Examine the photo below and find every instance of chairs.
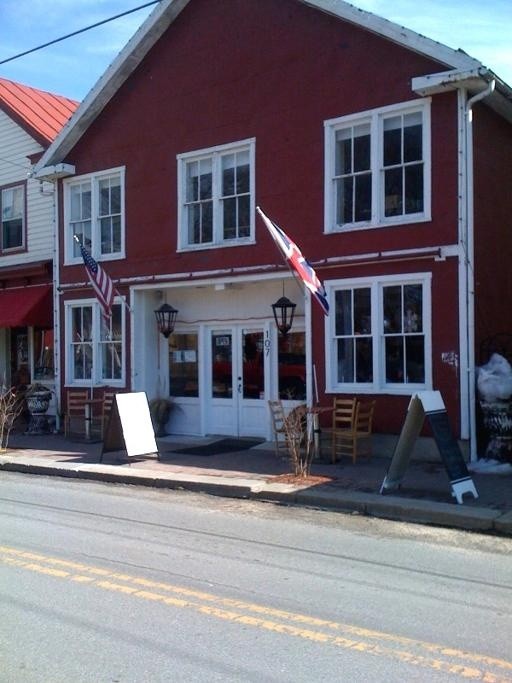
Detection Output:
[62,390,119,439]
[269,396,376,463]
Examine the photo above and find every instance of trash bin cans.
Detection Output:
[480,396,512,464]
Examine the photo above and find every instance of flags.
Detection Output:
[79,245,115,319]
[258,206,329,317]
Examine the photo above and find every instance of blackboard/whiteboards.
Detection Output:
[115,392,159,457]
[425,409,471,484]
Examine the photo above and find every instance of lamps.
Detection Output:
[272,293,295,332]
[155,302,178,337]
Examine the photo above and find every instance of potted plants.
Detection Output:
[150,399,176,438]
[24,382,52,434]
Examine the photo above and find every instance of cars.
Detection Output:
[213,345,306,399]
